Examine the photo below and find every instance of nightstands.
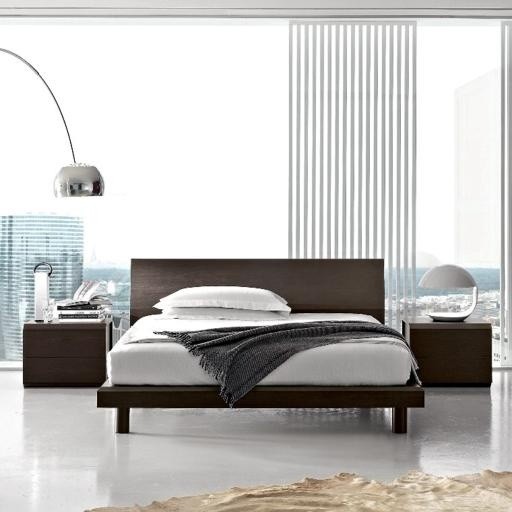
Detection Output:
[401,317,492,389]
[22,318,114,388]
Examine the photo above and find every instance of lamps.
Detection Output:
[0,48,104,199]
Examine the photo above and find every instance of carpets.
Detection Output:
[83,469,511,512]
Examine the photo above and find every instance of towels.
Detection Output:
[33,272,50,321]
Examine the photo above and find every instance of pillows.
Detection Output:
[151,285,293,322]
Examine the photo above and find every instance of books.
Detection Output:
[56,281,113,324]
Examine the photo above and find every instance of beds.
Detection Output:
[96,257,425,435]
